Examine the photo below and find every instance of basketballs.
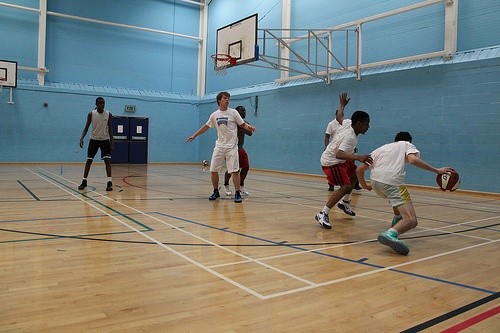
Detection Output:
[202,160,208,166]
[436,169,461,192]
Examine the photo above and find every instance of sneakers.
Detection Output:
[329,184,334,191]
[338,199,355,216]
[240,183,249,195]
[390,216,402,227]
[209,187,221,200]
[78,179,87,190]
[235,190,242,202]
[353,182,362,190]
[315,211,331,229]
[223,184,232,196]
[106,181,113,191]
[378,231,410,255]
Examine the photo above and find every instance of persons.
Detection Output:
[186,91,256,203]
[315,92,374,229]
[325,110,363,191]
[356,131,456,255]
[78,97,113,191]
[224,106,252,195]
